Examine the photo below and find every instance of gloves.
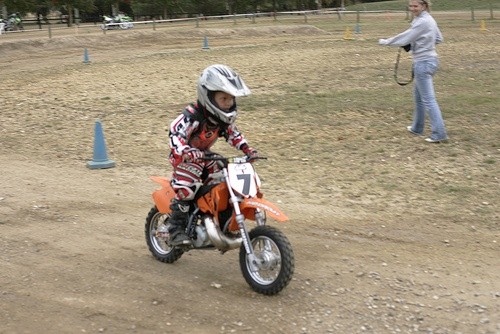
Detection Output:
[182,147,204,162]
[240,143,259,163]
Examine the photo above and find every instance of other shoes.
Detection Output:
[406,125,419,135]
[425,137,443,143]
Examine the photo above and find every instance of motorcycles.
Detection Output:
[145,152,294,296]
[2,17,24,32]
[101,12,133,30]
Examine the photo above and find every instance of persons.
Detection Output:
[169,64,259,246]
[378,0,450,142]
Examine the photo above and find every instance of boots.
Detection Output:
[167,199,192,246]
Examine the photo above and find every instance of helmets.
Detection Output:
[197,64,251,125]
